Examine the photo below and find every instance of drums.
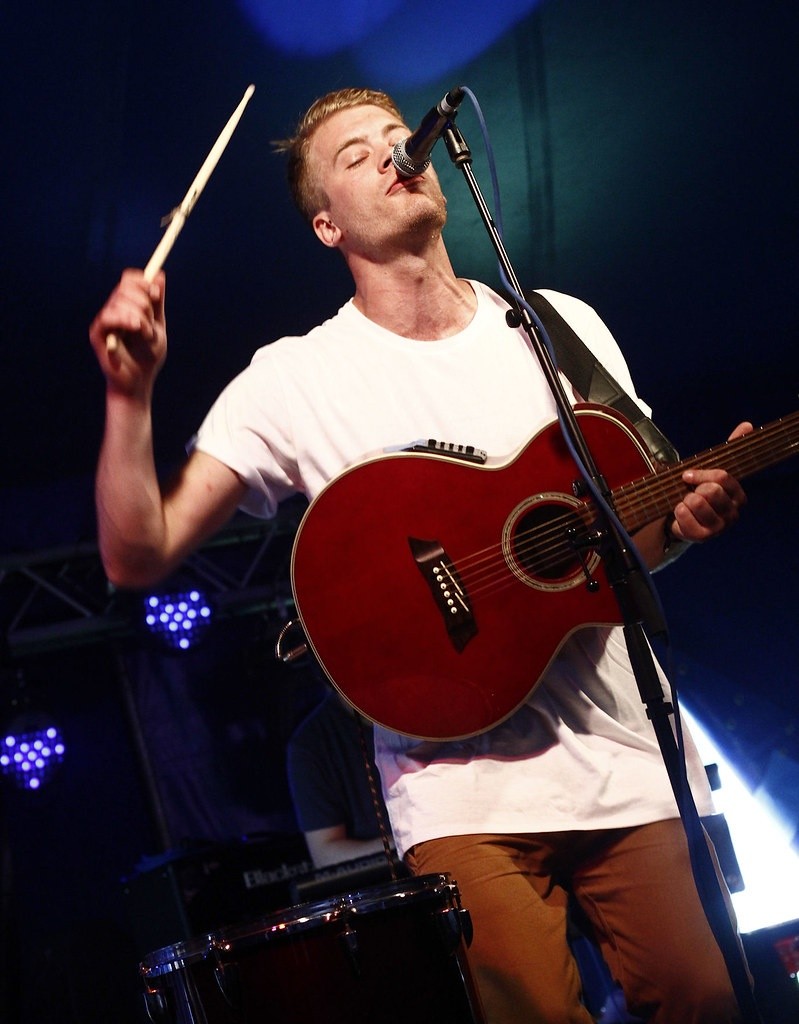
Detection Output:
[137,868,478,1023]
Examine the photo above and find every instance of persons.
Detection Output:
[87,88,754,1024]
[286,658,398,870]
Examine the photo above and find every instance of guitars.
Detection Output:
[288,398,799,743]
